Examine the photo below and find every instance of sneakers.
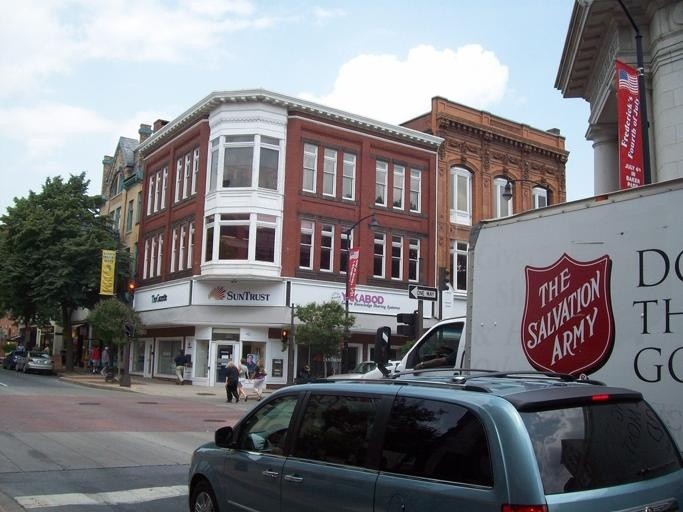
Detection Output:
[225,393,261,403]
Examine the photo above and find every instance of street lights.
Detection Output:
[341,211,377,373]
[502,177,551,208]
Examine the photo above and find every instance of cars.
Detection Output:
[348,360,400,374]
[2,350,24,369]
[15,350,55,374]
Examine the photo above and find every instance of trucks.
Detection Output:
[361,178,683,458]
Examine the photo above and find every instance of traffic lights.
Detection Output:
[127,279,135,298]
[438,265,449,293]
[125,324,133,338]
[395,311,416,335]
[280,330,286,341]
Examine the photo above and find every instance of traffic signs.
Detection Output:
[406,283,438,301]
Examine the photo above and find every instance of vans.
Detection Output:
[185,374,681,511]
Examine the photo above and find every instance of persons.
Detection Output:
[89,345,100,374]
[250,358,267,401]
[172,349,187,385]
[237,358,249,402]
[246,354,256,374]
[43,342,50,352]
[297,365,310,383]
[15,342,25,352]
[222,360,238,403]
[100,346,111,375]
